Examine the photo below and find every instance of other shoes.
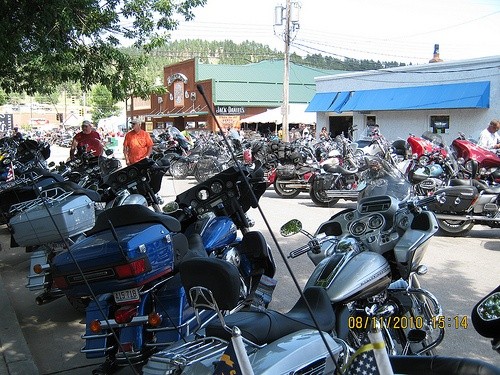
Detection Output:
[157,196,164,205]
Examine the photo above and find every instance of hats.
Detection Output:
[81,120,90,127]
[129,119,142,125]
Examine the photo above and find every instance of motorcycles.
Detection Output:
[0,127,500,375]
[51,160,278,375]
[143,155,446,375]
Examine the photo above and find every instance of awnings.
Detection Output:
[304,80,490,113]
[240,102,317,125]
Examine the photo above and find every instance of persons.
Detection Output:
[5,129,41,139]
[476,119,500,154]
[278,123,327,141]
[70,120,103,160]
[123,118,153,165]
